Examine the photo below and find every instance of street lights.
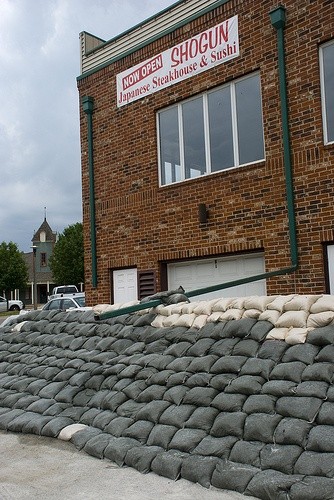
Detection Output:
[30,245,43,309]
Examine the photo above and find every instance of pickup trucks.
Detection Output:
[47,285,84,302]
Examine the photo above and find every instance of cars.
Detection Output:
[42,295,85,310]
[0,295,23,311]
